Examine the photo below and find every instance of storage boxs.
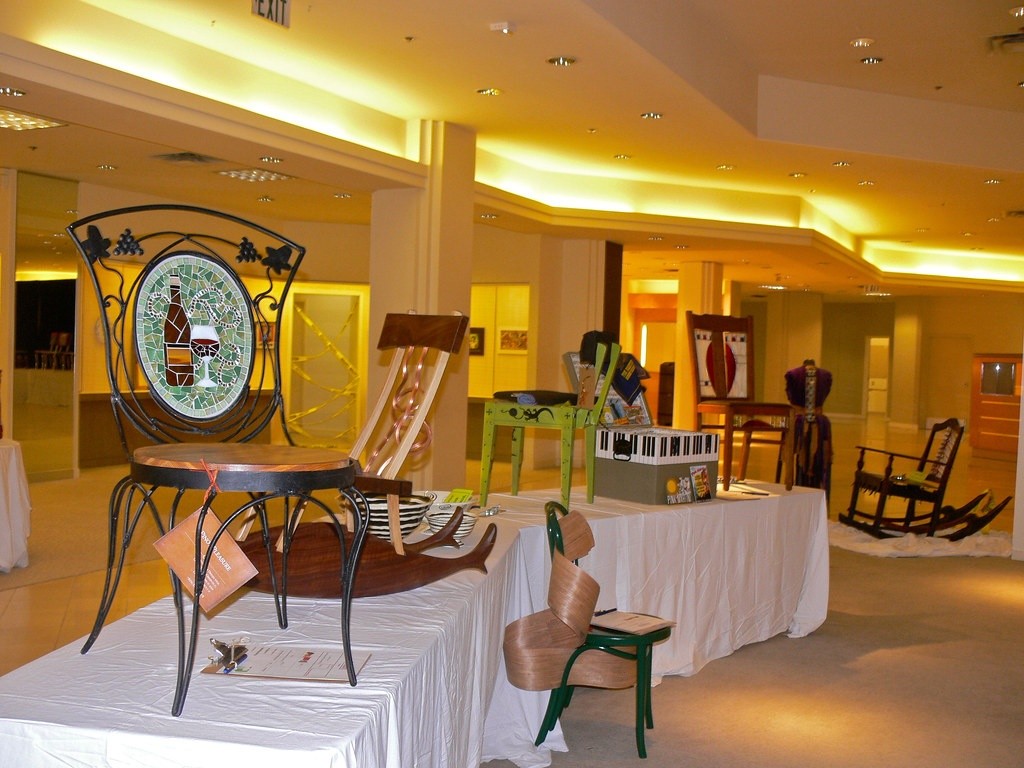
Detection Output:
[563,351,718,504]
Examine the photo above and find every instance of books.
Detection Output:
[690,465,712,504]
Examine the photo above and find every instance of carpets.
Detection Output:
[828,519,1014,557]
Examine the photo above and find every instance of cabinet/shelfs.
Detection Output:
[968,353,1023,463]
[658,362,673,425]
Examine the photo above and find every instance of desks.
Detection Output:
[13,368,73,409]
[0,436,831,768]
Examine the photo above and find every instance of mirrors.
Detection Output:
[981,362,1016,395]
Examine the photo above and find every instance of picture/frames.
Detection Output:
[470,327,485,355]
[254,321,275,349]
[497,327,528,355]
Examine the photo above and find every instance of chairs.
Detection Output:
[534,499,670,759]
[479,342,621,507]
[841,420,1013,540]
[66,204,371,722]
[227,308,498,601]
[34,331,73,369]
[685,309,798,490]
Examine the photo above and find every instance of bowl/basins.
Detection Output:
[425,511,478,548]
[346,493,436,542]
[412,491,479,517]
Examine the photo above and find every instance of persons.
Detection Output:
[784,359,832,487]
